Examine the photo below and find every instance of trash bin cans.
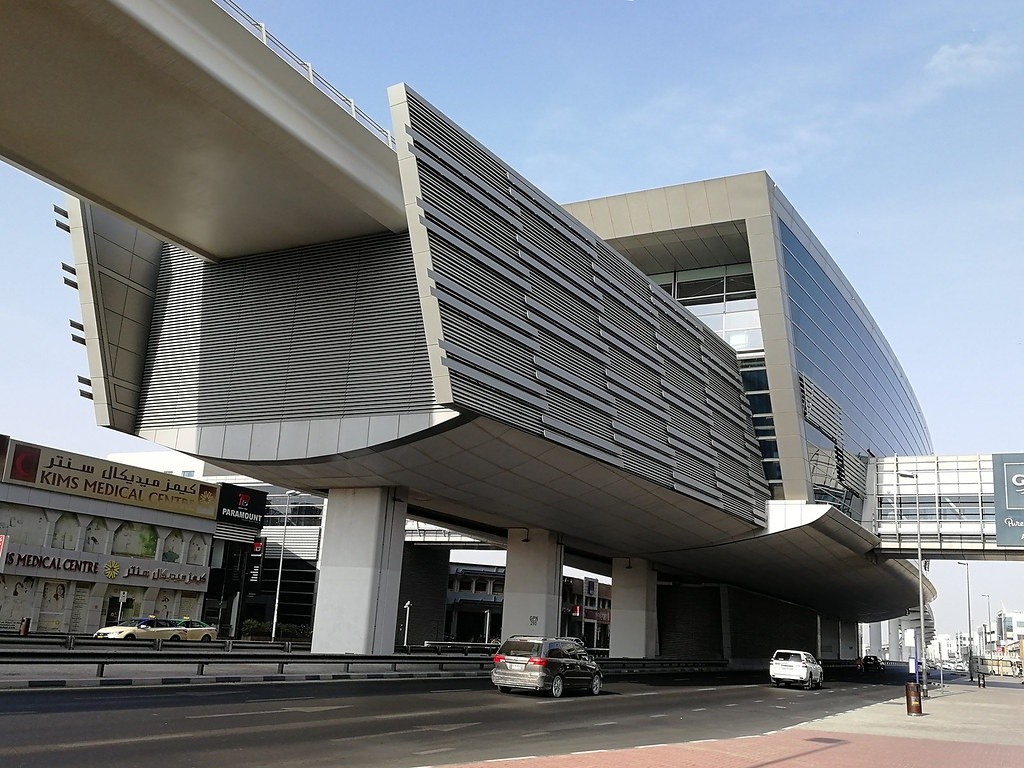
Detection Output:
[978,674,985,688]
[906,683,922,716]
[19,617,31,635]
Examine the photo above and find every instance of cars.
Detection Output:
[862,655,886,673]
[918,658,969,676]
[92,614,189,642]
[171,616,218,643]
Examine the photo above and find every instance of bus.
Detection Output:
[969,653,1023,678]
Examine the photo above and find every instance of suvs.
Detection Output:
[489,634,604,698]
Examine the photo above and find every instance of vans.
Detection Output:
[768,649,824,690]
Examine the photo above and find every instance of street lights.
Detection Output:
[269,489,301,641]
[894,470,929,700]
[957,561,973,682]
[982,594,994,676]
